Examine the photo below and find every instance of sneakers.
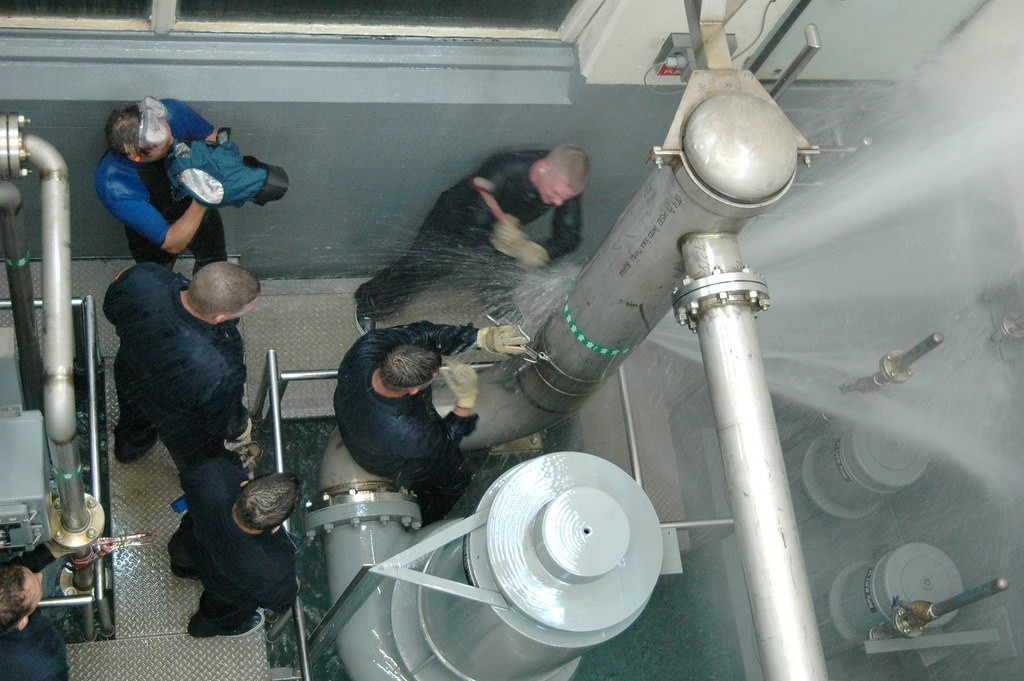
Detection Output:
[232,441,262,473]
[355,301,377,335]
[214,610,265,637]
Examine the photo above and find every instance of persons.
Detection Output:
[334,320,527,527]
[95,98,228,276]
[0,540,74,681]
[355,144,590,335]
[103,262,261,468]
[169,454,301,638]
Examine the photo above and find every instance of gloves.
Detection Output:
[477,325,526,356]
[442,359,479,408]
[44,536,75,558]
[491,212,549,271]
[224,417,252,452]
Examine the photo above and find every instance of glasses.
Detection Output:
[137,95,169,154]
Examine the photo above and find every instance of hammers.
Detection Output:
[471,175,508,223]
